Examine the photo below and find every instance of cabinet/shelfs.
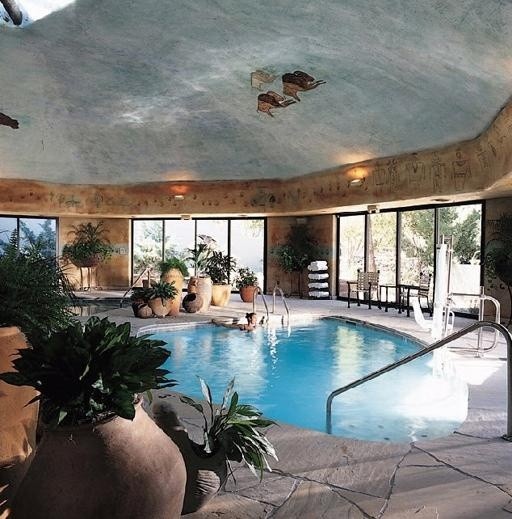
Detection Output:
[307,267,329,300]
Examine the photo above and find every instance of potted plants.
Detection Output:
[203,250,236,307]
[234,267,259,302]
[0,315,187,519]
[142,372,280,515]
[129,287,150,318]
[0,228,98,465]
[157,256,189,317]
[182,233,218,313]
[139,279,178,317]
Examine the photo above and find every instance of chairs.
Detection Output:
[347,269,382,309]
[400,272,432,317]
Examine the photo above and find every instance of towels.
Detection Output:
[307,260,330,299]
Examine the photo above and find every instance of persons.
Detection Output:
[211,311,260,333]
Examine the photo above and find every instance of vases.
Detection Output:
[183,292,203,312]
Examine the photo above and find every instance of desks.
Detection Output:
[379,285,418,314]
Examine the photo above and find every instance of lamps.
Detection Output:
[169,185,187,202]
[345,166,368,189]
